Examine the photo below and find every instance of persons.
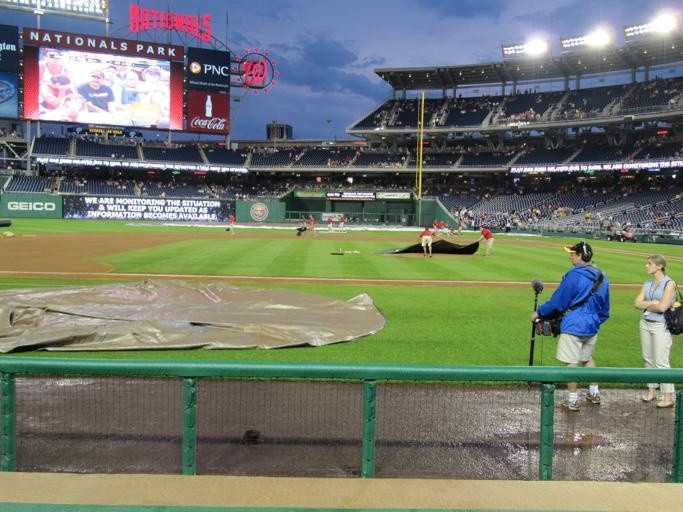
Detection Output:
[36,48,169,126]
[373,74,683,126]
[477,225,494,255]
[634,255,677,407]
[531,241,610,411]
[419,226,434,257]
[0,127,683,242]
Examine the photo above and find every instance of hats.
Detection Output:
[90,70,104,78]
[564,242,590,254]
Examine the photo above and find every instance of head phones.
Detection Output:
[581,242,591,262]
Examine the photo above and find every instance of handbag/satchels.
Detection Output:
[665,299,683,335]
[550,310,563,334]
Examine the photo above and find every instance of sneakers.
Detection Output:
[562,393,601,410]
[643,391,675,407]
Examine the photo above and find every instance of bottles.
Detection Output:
[205,95,212,117]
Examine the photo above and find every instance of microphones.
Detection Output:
[531,279,544,294]
